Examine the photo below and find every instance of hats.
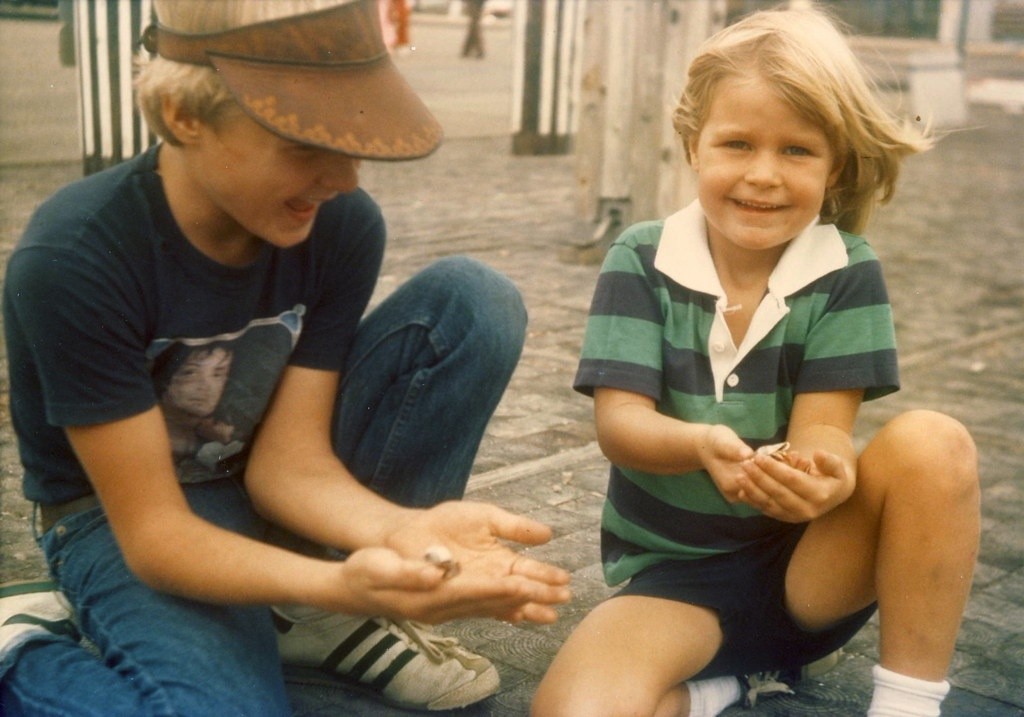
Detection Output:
[142,0,443,160]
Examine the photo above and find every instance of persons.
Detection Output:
[383,0,412,49]
[528,0,982,717]
[2,0,571,717]
[458,0,487,59]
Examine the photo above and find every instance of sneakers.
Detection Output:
[269,598,502,714]
[0,580,84,675]
[729,649,841,709]
